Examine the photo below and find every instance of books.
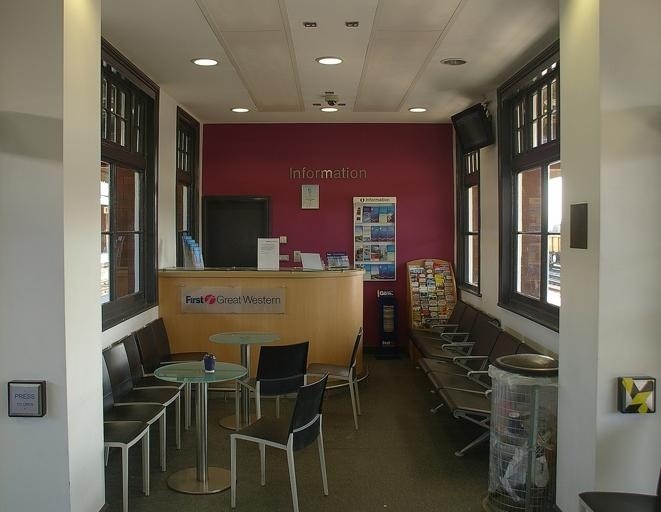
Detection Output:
[298,251,323,272]
[354,204,396,280]
[179,229,202,266]
[325,250,350,270]
[409,260,456,329]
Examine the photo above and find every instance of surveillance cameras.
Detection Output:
[326,96,342,106]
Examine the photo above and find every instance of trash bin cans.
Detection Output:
[482,354,559,512]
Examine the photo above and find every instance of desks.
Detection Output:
[207,331,279,430]
[151,361,248,495]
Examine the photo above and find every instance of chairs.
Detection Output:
[103,319,208,511]
[577,458,661,512]
[241,338,308,418]
[228,371,332,510]
[304,329,367,429]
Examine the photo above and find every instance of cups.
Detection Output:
[202,352,217,374]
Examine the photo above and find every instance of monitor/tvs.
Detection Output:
[450,102,495,153]
[202,194,271,266]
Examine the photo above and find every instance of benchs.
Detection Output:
[408,297,501,396]
[417,321,547,458]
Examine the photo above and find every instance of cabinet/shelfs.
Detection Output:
[407,257,458,330]
